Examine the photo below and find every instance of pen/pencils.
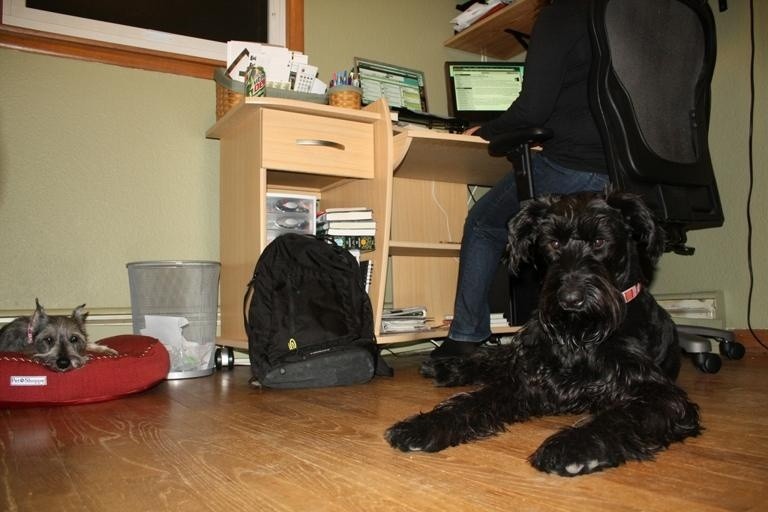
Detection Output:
[330,68,360,87]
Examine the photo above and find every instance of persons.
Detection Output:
[428,0,611,366]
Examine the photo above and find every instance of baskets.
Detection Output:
[328,85,361,110]
[213,66,327,121]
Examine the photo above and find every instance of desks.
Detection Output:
[377,120,543,347]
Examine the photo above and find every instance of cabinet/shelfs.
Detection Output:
[204,92,396,351]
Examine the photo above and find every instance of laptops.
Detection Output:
[354,56,461,123]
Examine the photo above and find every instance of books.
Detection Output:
[315,204,380,250]
[383,304,511,337]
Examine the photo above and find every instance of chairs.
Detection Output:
[489,1,746,375]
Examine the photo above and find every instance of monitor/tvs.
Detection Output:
[444,61,526,128]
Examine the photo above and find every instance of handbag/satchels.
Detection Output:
[243,232,393,389]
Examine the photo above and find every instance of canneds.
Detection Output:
[246,66,266,97]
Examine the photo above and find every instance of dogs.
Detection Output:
[384,182,706,477]
[0,299,120,373]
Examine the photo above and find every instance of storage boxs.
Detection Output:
[263,191,319,252]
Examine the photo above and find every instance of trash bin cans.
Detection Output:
[126,263,218,381]
